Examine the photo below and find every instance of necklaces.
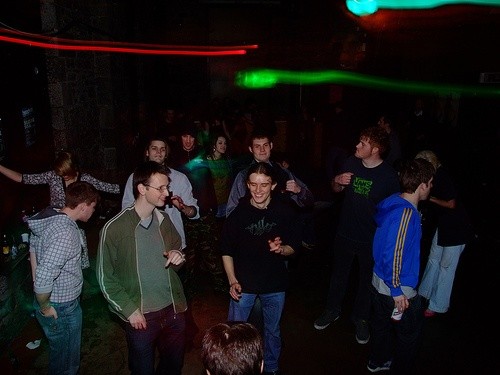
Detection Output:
[251,198,267,210]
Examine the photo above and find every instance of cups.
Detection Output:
[20,233,29,242]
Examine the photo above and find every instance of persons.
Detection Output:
[377,116,402,165]
[120,136,200,252]
[226,131,315,332]
[0,151,120,270]
[153,108,185,148]
[170,127,234,309]
[96,161,189,375]
[219,162,305,375]
[313,126,402,345]
[27,180,101,375]
[413,150,471,318]
[201,321,264,375]
[366,157,436,373]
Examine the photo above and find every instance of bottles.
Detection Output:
[11,233,17,260]
[391,307,402,321]
[2,234,10,257]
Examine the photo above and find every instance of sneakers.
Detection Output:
[355,319,371,344]
[366,357,394,372]
[313,310,341,330]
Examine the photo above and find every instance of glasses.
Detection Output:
[247,182,269,191]
[144,184,171,193]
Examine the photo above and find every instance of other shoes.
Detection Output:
[205,276,230,296]
[422,302,427,306]
[179,275,193,301]
[423,309,436,319]
